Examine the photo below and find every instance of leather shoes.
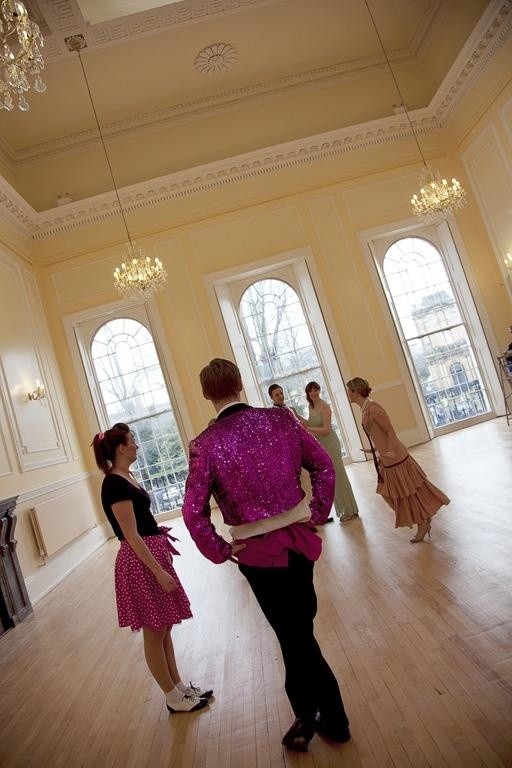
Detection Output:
[328,517,333,522]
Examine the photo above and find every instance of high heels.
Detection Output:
[409,524,431,543]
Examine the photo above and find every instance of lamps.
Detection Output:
[362,1,468,227]
[64,34,170,303]
[28,378,46,403]
[0,0,49,114]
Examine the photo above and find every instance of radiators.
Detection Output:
[29,485,97,557]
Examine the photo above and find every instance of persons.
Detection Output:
[182,359,351,751]
[268,384,333,523]
[290,382,358,522]
[346,377,450,543]
[93,423,213,712]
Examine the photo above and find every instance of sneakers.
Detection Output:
[282,718,316,751]
[315,713,350,744]
[166,695,208,712]
[185,687,213,699]
